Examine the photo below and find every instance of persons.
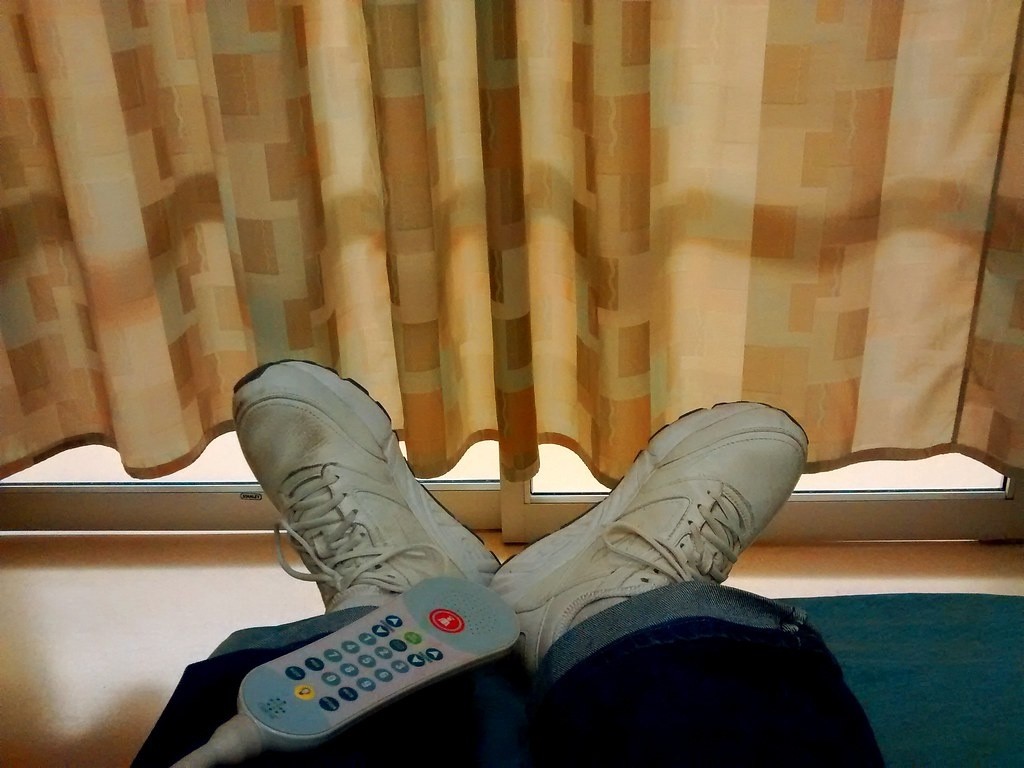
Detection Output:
[130,359,885,768]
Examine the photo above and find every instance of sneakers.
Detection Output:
[490,397,812,683]
[231,357,500,602]
